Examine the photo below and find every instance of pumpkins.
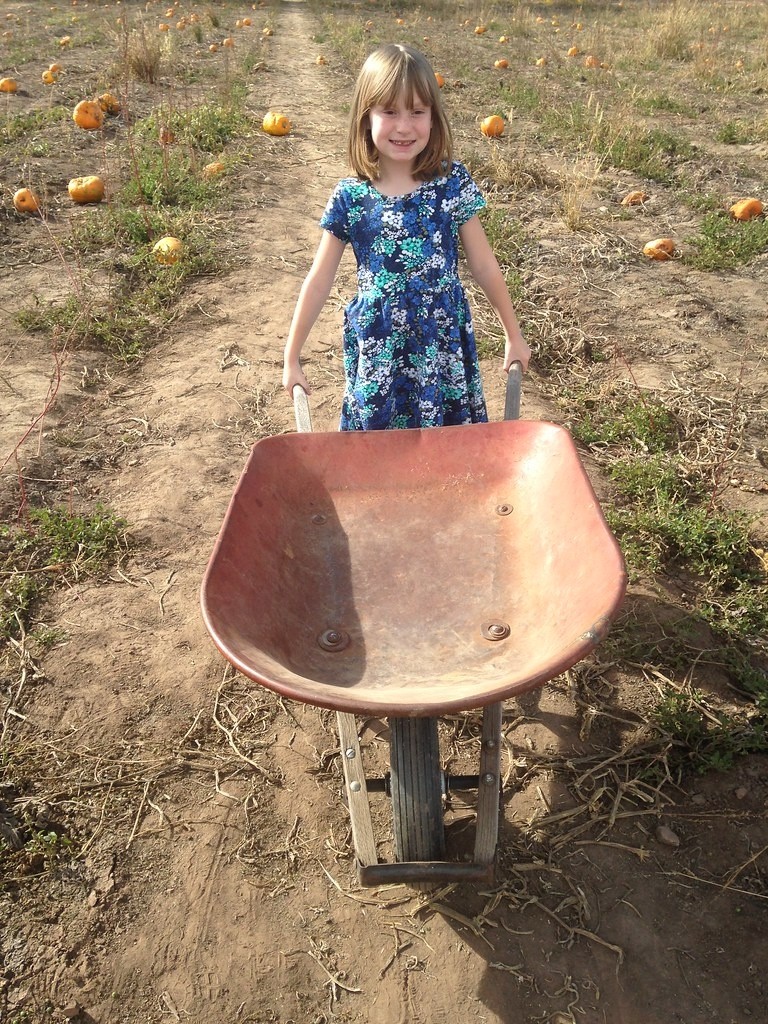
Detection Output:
[0,0,763,264]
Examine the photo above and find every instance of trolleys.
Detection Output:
[196,353,636,891]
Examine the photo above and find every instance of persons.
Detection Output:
[281,44,532,431]
